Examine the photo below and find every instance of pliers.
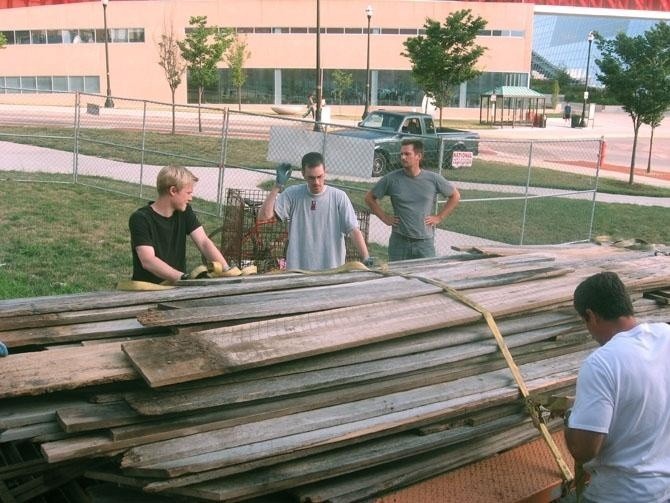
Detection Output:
[513,394,574,417]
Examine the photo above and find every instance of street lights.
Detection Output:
[362,4,374,119]
[580,29,595,127]
[100,0,114,109]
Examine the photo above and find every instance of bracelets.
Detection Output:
[563,406,574,423]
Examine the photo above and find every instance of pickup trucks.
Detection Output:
[327,109,481,178]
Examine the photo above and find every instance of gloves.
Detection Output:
[361,256,374,266]
[180,273,210,280]
[276,162,292,188]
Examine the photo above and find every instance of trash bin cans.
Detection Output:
[571,114,580,127]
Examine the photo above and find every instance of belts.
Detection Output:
[392,232,424,241]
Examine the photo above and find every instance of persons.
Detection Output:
[563,100,572,128]
[302,92,315,120]
[561,272,670,503]
[365,138,460,261]
[257,151,373,273]
[128,162,230,285]
